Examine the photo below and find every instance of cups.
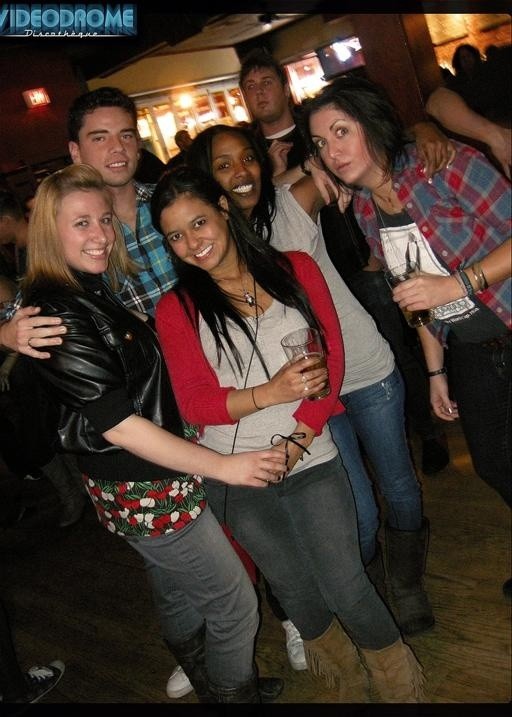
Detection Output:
[280,327,332,402]
[385,260,436,329]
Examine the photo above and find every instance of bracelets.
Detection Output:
[250,384,264,409]
[458,271,474,297]
[479,267,489,290]
[425,368,446,377]
[451,272,466,298]
[471,265,483,291]
[270,432,309,462]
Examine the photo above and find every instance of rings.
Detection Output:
[303,382,308,390]
[299,372,306,381]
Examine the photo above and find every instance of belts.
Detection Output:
[471,331,511,352]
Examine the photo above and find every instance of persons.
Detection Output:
[162,36,511,176]
[0,631,73,705]
[181,117,457,637]
[18,162,294,707]
[240,51,450,478]
[2,86,355,699]
[144,165,434,705]
[306,74,512,602]
[0,166,91,530]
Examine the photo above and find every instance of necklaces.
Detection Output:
[219,270,263,307]
[367,184,400,214]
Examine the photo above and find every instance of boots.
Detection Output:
[39,450,90,530]
[163,618,284,704]
[356,637,428,704]
[300,614,371,704]
[384,514,436,638]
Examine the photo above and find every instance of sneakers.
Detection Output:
[280,617,309,671]
[166,663,194,699]
[22,658,67,704]
[421,428,450,473]
